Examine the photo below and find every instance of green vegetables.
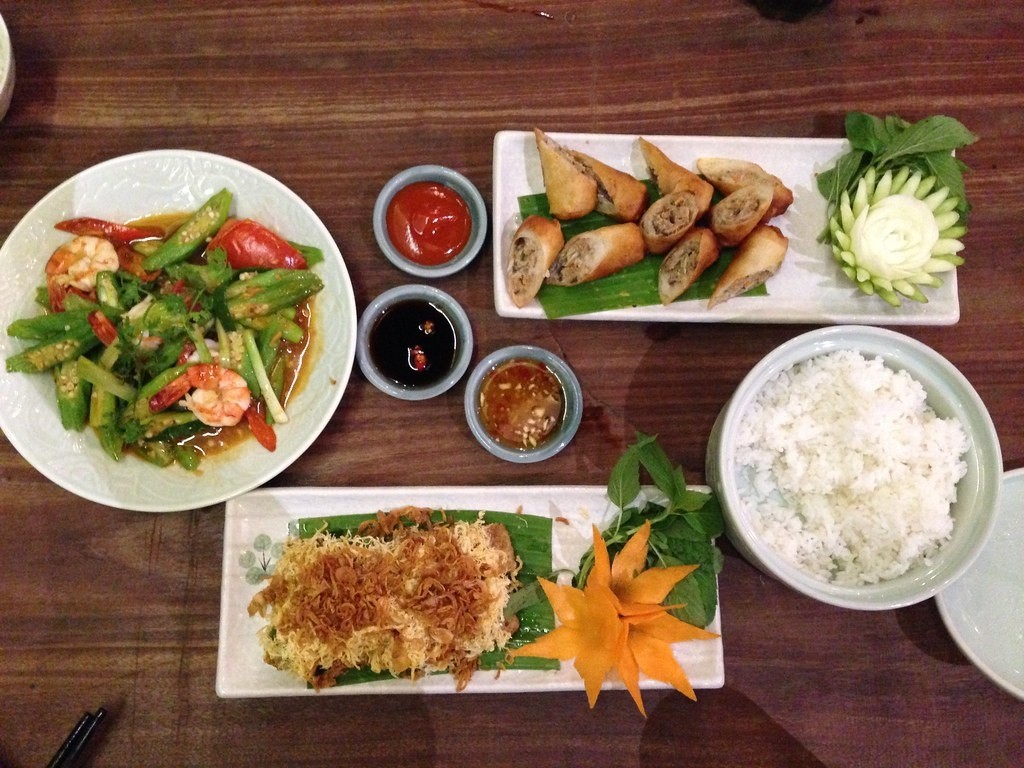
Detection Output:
[816,111,975,247]
[575,433,726,631]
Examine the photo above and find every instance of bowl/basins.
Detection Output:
[705,325,1003,609]
[357,285,473,399]
[374,165,487,276]
[463,346,584,463]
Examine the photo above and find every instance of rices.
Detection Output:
[730,348,971,587]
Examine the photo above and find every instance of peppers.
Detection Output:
[2,187,326,470]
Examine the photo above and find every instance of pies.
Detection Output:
[507,127,795,312]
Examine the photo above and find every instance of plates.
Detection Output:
[217,484,726,696]
[1,149,358,512]
[492,131,959,326]
[934,468,1023,700]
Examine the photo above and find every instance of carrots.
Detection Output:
[508,519,723,718]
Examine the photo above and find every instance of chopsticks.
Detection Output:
[46,707,105,768]
